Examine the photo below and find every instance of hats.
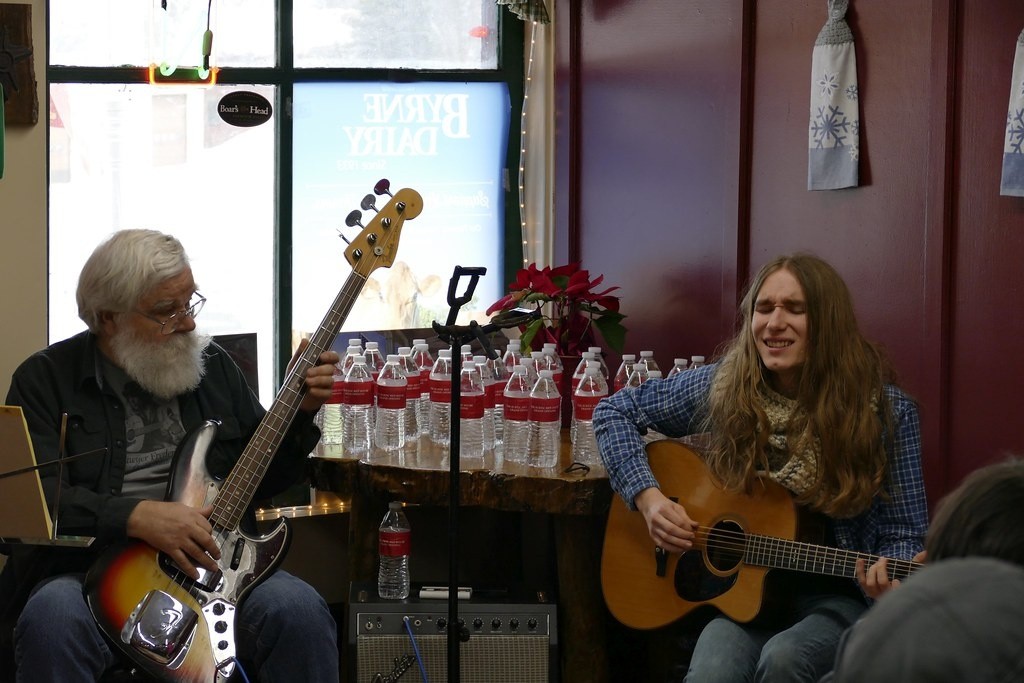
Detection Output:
[836,558,1024,683]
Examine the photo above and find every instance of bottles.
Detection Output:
[378,502,410,599]
[300,331,708,466]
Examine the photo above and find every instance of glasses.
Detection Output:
[135,292,207,335]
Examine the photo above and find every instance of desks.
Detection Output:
[304,426,613,683]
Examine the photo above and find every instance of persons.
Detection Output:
[592,252,928,683]
[5,229,340,683]
[830,458,1024,683]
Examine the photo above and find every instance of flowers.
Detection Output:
[487,263,630,355]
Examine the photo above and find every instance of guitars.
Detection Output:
[601,439,929,633]
[83,177,424,683]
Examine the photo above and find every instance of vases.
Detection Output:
[562,351,607,426]
